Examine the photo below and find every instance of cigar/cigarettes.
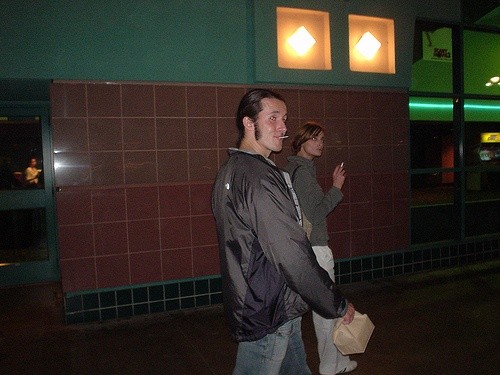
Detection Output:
[340,162,344,167]
[279,136,288,138]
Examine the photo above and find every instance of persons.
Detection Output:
[285,122,357,375]
[25,159,42,190]
[211,89,355,375]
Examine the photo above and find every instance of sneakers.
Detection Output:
[320,360,357,375]
